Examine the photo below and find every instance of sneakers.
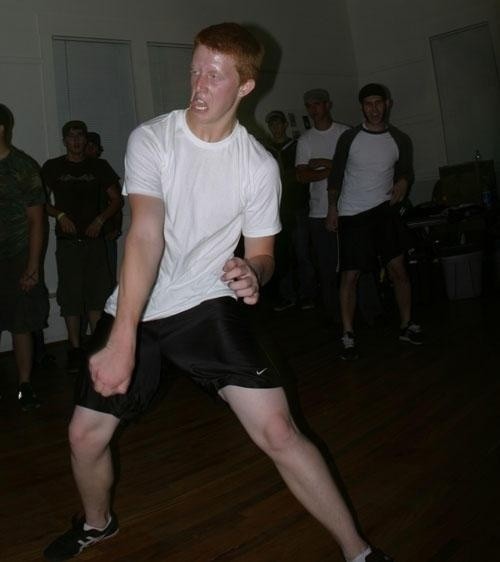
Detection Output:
[399,321,424,345]
[341,331,358,361]
[43,507,120,562]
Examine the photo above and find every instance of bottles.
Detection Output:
[479,175,492,209]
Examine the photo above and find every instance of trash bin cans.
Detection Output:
[438,245,485,304]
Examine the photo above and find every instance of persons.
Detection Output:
[39,121,122,369]
[83,130,125,286]
[327,84,433,365]
[43,23,402,561]
[1,101,49,403]
[291,85,397,338]
[264,104,316,312]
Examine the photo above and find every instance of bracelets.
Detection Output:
[97,216,102,225]
[56,211,64,219]
